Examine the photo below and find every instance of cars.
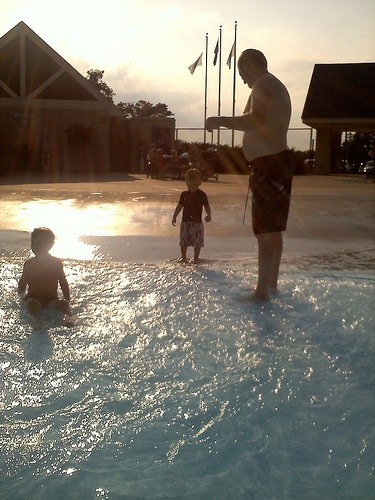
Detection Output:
[305,156,375,177]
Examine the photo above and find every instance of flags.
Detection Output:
[211,37,219,66]
[226,40,235,69]
[188,51,203,74]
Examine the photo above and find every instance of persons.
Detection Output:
[205,47,294,307]
[15,225,76,330]
[141,147,213,182]
[170,166,213,266]
